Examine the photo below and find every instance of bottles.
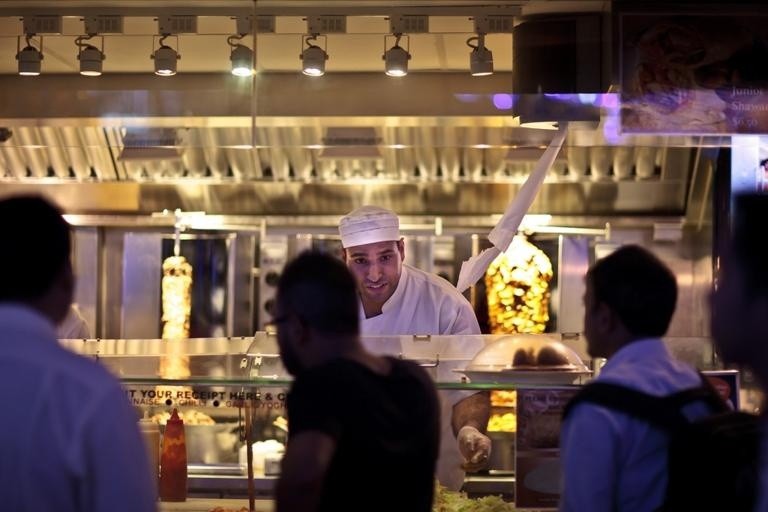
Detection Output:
[136,405,189,506]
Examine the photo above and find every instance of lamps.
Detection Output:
[505,127,570,163]
[317,126,383,160]
[114,127,184,162]
[13,26,494,79]
[152,225,202,406]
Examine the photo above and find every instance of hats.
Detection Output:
[339,206,401,248]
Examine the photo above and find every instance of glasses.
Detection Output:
[263,320,284,337]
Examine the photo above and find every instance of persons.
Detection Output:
[339,205,491,491]
[274,250,439,512]
[1,192,157,511]
[53,306,89,339]
[558,243,729,512]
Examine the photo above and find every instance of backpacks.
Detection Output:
[562,370,762,512]
